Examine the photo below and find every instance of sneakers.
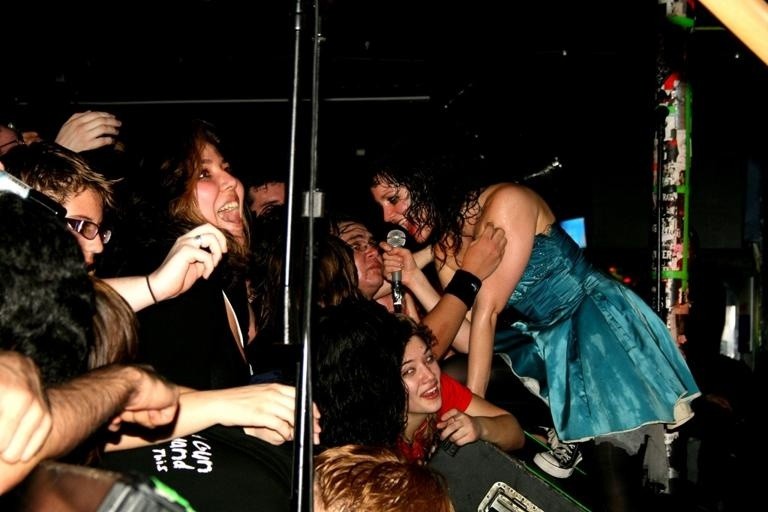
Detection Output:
[665,429,679,479]
[534,428,583,479]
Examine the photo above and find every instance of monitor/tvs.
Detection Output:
[559,217,587,249]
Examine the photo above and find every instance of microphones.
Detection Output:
[386,229,407,314]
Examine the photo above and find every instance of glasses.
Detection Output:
[0,138,24,147]
[66,217,112,245]
[351,240,379,254]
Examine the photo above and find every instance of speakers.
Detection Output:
[422,435,611,512]
